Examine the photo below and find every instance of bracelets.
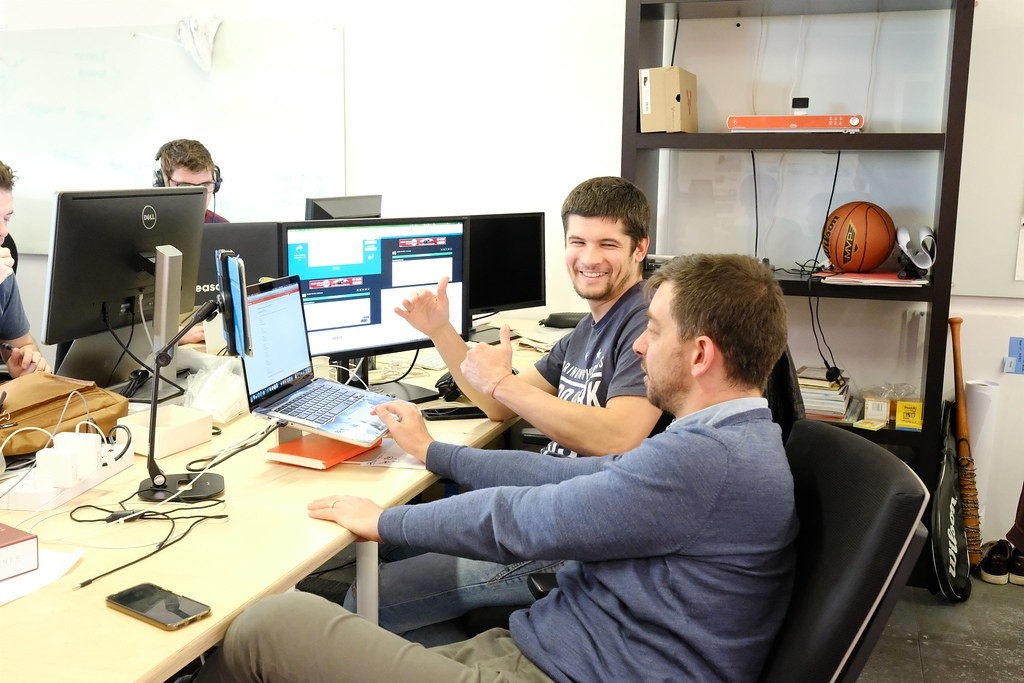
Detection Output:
[492,373,512,399]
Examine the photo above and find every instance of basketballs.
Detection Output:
[822,201,897,272]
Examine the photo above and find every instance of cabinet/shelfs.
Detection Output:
[619,0,977,584]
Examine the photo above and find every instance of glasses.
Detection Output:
[166,174,216,193]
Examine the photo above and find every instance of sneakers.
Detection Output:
[979,538,1024,586]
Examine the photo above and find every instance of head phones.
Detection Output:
[151,142,222,194]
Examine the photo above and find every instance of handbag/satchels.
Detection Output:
[0,369,129,456]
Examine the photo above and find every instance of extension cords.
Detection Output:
[0,444,135,511]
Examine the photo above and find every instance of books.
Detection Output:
[796,365,864,424]
[267,432,383,470]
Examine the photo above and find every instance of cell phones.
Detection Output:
[105,582,211,631]
[421,406,488,421]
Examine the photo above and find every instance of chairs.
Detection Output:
[756,420,932,683]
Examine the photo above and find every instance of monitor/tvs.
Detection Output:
[40,186,548,405]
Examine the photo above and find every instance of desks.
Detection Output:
[0,308,582,682]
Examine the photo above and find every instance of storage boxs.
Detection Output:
[639,68,697,132]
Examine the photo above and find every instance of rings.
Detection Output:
[330,500,341,508]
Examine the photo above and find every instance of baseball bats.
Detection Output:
[948,315,983,565]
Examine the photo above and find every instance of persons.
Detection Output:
[153,139,231,345]
[342,175,675,635]
[0,163,52,379]
[174,253,800,683]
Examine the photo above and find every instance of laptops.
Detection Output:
[56,319,154,388]
[243,274,409,447]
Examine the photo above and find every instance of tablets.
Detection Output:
[213,248,256,360]
